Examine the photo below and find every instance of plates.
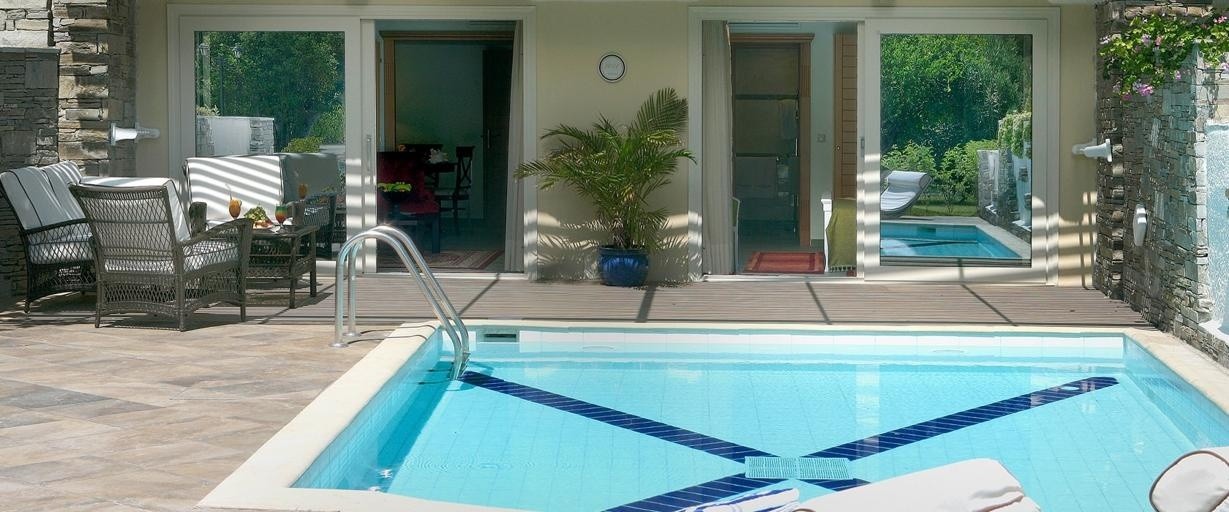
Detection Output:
[253,224,274,230]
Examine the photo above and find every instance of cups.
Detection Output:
[298,184,307,201]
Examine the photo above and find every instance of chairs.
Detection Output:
[879,170,933,219]
[70,155,286,331]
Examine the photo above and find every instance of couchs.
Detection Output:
[0,160,98,312]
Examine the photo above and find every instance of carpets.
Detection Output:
[742,251,825,274]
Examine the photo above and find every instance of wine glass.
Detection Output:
[229,199,241,220]
[275,204,288,232]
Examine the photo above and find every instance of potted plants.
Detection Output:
[513,88,699,286]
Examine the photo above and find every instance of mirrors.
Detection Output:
[878,34,1033,268]
